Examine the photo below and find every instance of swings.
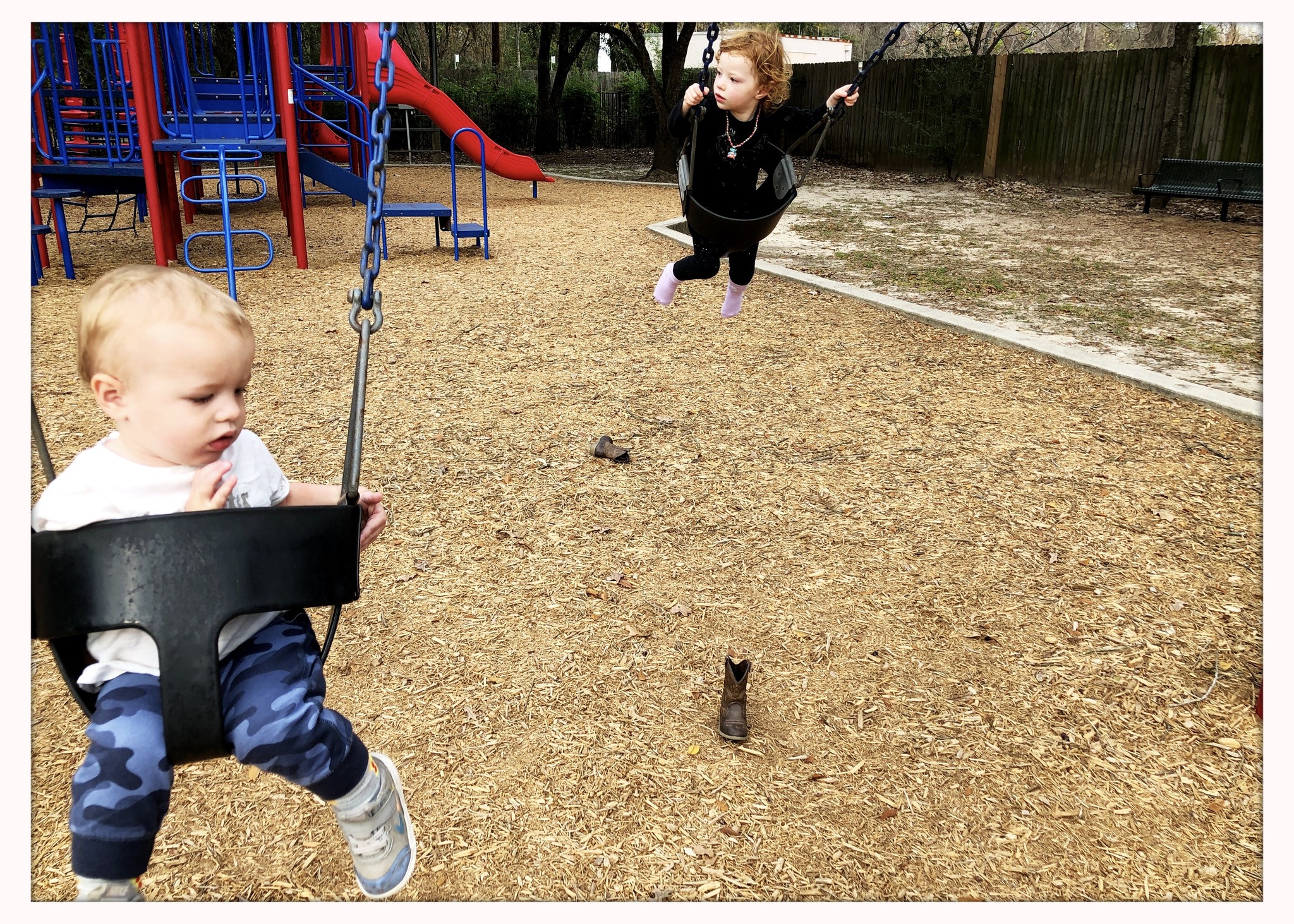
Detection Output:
[676,21,910,252]
[33,23,399,763]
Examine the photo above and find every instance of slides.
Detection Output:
[292,23,557,182]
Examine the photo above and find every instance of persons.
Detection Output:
[654,22,859,319]
[31,265,417,902]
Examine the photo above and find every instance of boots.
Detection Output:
[718,655,752,741]
[590,435,631,463]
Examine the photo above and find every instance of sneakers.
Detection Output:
[76,880,146,902]
[331,752,416,898]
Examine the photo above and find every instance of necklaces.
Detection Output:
[726,105,761,160]
